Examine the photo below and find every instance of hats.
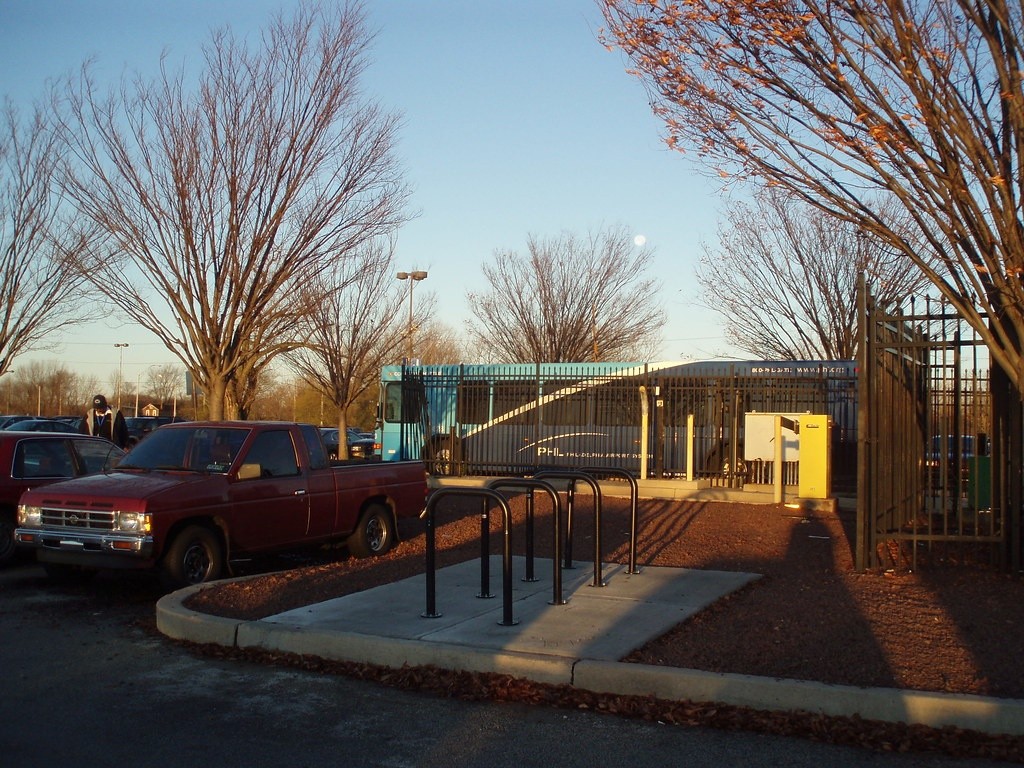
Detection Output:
[92,394,107,408]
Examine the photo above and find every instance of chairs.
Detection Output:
[36,457,70,477]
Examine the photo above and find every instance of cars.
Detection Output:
[1,432,130,562]
[926,435,975,480]
[0,414,186,452]
[319,427,374,461]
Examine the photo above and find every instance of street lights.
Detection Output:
[396,269,429,365]
[114,343,129,410]
[134,364,162,417]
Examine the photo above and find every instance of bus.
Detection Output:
[368,353,860,485]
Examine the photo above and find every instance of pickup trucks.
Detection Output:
[13,420,430,594]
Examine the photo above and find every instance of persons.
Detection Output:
[78,394,129,453]
